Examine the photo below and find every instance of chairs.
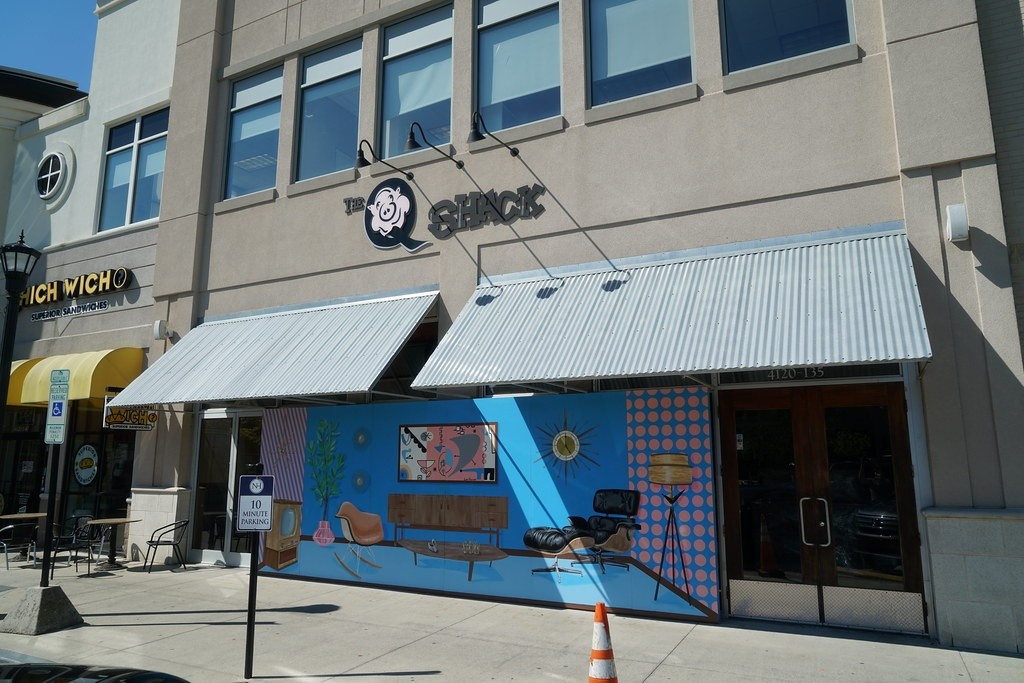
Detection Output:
[144,519,189,573]
[50,515,109,578]
[0,523,39,570]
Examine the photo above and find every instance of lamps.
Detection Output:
[354,140,413,180]
[467,112,519,157]
[405,122,464,169]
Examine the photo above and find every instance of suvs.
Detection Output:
[831,454,901,574]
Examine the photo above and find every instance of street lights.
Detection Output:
[0,229,44,421]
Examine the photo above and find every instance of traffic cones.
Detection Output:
[586,599,620,683]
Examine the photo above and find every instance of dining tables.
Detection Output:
[88,518,142,570]
[0,513,47,561]
